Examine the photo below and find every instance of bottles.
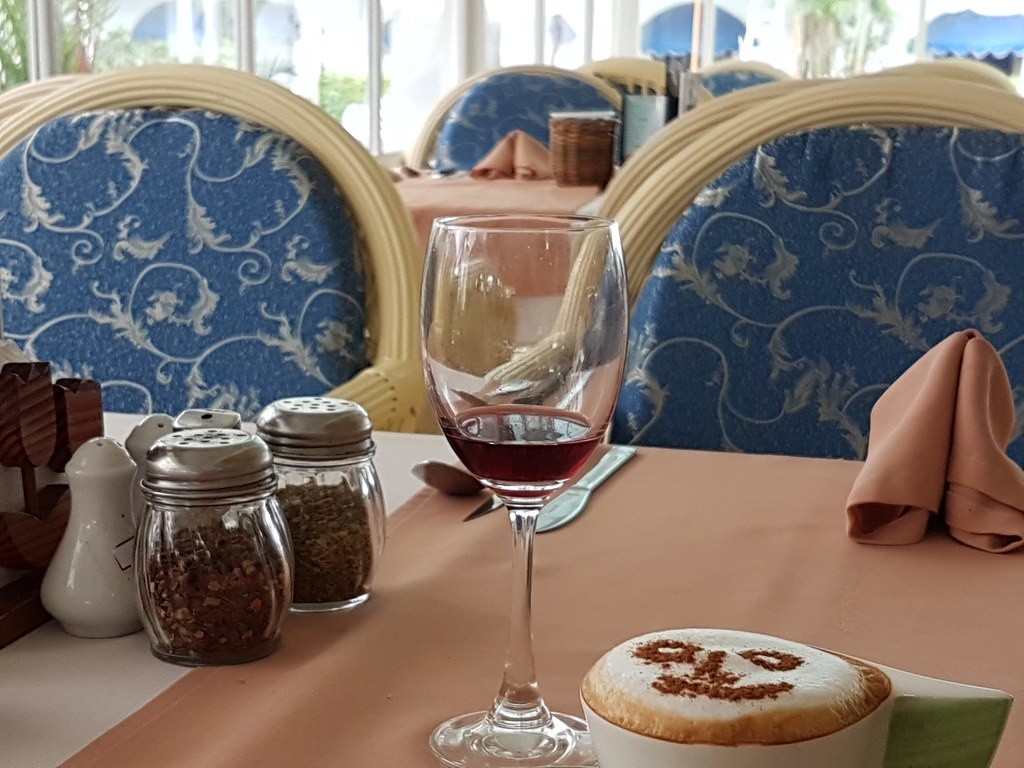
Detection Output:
[39,396,387,668]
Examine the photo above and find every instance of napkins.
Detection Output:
[846,332,1024,555]
[472,130,553,182]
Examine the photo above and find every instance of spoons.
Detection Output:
[412,460,488,495]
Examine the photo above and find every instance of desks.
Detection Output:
[395,171,601,339]
[0,412,1024,768]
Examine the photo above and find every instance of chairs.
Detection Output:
[409,57,1024,464]
[0,63,442,437]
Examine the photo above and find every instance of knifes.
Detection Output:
[534,445,639,533]
[460,492,506,522]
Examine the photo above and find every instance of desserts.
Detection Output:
[579,628,893,768]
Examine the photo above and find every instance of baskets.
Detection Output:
[550,116,614,187]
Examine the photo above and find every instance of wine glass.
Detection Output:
[421,213,629,766]
